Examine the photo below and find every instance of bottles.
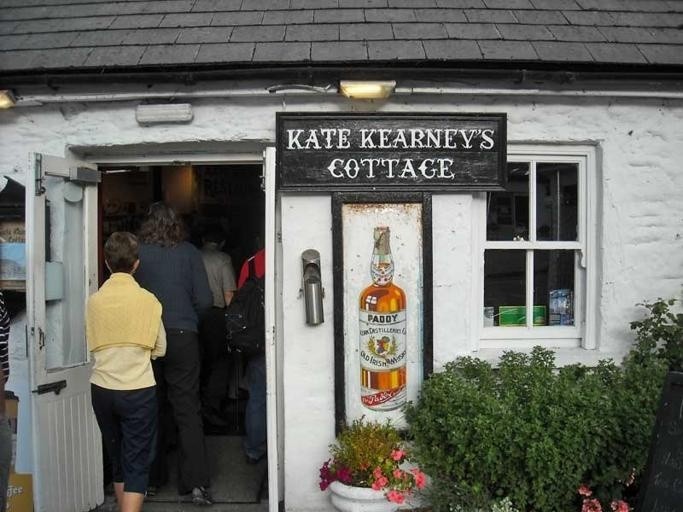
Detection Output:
[357,226,409,412]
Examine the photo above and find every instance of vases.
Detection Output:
[328,480,402,512]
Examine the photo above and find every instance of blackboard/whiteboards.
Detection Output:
[632,371,683,512]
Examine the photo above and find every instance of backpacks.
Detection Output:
[224,256,265,354]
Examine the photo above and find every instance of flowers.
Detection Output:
[319,413,426,504]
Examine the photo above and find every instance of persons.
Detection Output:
[190,212,266,467]
[0,297,12,511]
[83,227,169,511]
[132,195,215,507]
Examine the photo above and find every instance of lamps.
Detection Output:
[0,87,20,109]
[337,76,397,100]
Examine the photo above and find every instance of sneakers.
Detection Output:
[247,455,263,464]
[148,487,160,495]
[192,487,214,505]
[205,407,227,426]
